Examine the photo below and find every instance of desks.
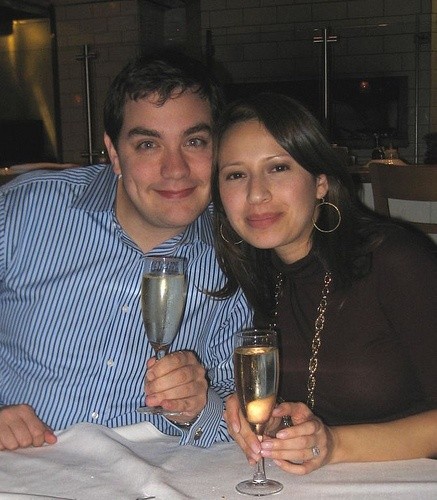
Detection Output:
[361,183,437,245]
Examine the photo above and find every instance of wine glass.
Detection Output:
[234,328,284,496]
[136,256,186,415]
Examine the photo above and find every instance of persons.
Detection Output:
[0,53,252,455]
[211,91,436,475]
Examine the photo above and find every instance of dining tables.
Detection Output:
[0,421,437,499]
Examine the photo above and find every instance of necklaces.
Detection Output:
[268,258,334,432]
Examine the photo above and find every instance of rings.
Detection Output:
[311,445,320,459]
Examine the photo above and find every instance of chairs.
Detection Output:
[369,162,437,234]
